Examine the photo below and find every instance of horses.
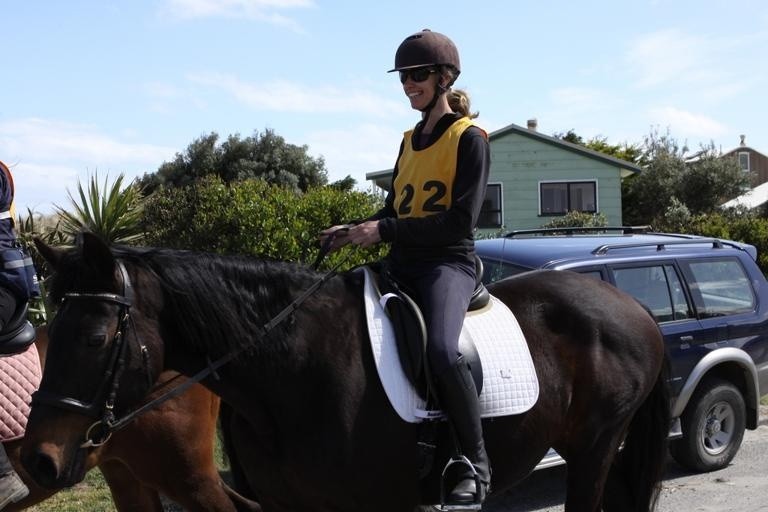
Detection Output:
[0,228,671,512]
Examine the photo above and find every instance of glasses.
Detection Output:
[399,68,437,84]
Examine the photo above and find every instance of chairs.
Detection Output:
[643,279,678,310]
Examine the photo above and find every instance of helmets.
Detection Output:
[387,29,461,73]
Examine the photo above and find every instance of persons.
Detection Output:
[319,28,493,505]
[0,160,42,511]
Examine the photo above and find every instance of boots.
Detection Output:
[0,444,29,510]
[428,352,492,504]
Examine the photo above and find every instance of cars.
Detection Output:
[475,226,768,472]
